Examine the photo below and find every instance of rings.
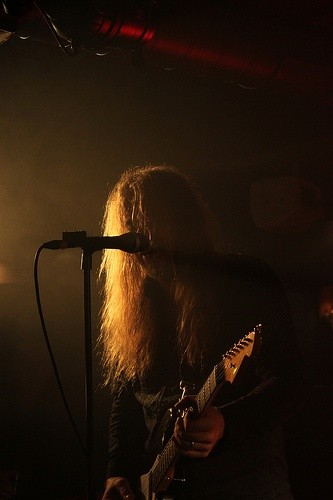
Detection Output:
[190,442,196,449]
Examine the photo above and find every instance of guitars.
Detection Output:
[119,320,266,498]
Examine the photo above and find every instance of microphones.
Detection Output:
[43,232,149,254]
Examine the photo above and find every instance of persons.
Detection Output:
[94,163,299,500]
